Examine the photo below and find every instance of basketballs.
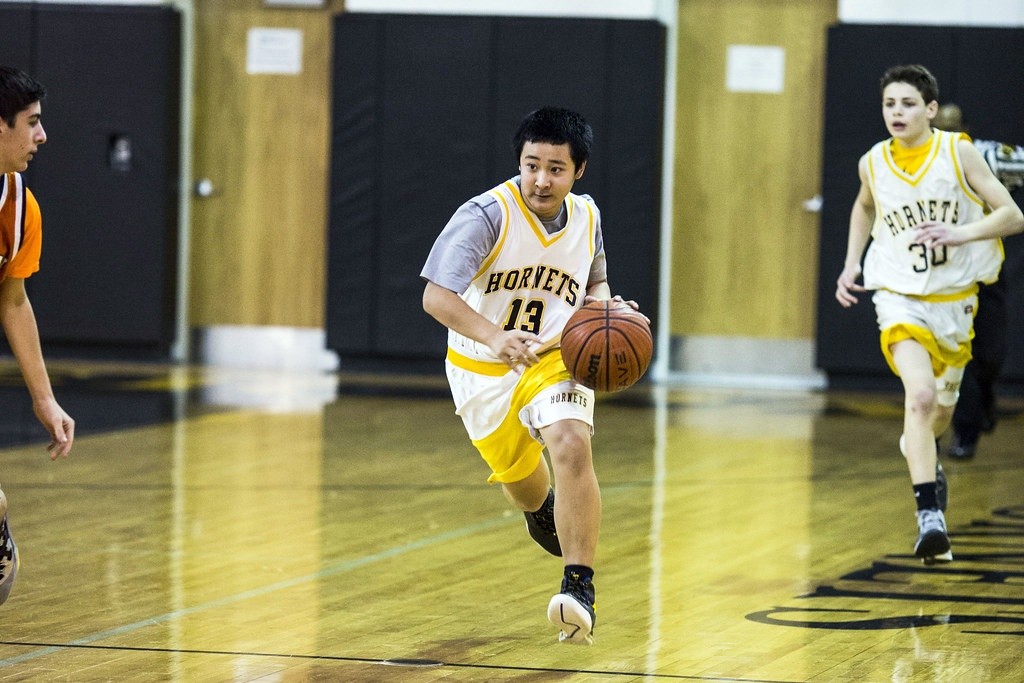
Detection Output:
[560,299,654,393]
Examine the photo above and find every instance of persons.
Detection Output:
[0,68,74,609]
[835,64,1024,567]
[932,103,1024,457]
[419,107,650,645]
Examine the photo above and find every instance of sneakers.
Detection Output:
[913,508,953,565]
[524,485,563,557]
[900,433,946,513]
[546,572,595,644]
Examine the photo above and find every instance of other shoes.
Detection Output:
[950,435,978,458]
[0,512,20,606]
